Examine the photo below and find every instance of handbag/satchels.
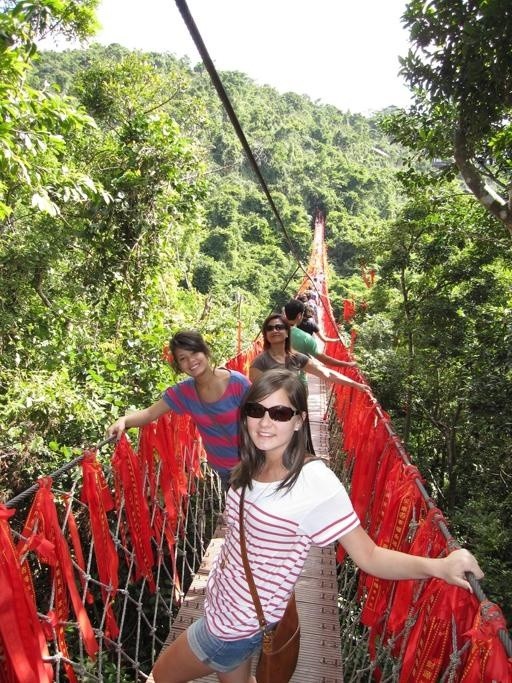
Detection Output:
[256,590,300,683]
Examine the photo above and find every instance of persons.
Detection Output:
[248,313,373,392]
[282,298,361,400]
[152,369,484,683]
[297,302,340,343]
[298,257,325,306]
[105,329,256,492]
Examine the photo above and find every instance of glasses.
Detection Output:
[266,324,286,331]
[243,402,301,422]
